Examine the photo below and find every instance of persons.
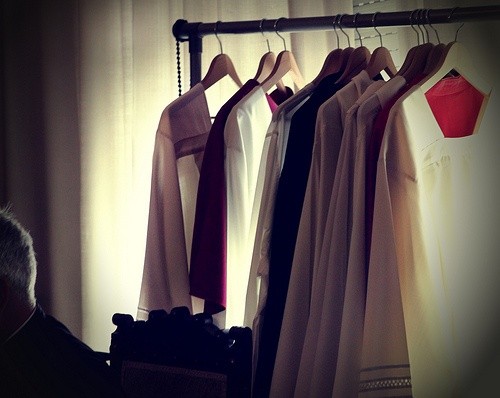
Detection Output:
[0,210,126,398]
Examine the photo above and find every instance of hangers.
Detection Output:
[164,9,500,121]
